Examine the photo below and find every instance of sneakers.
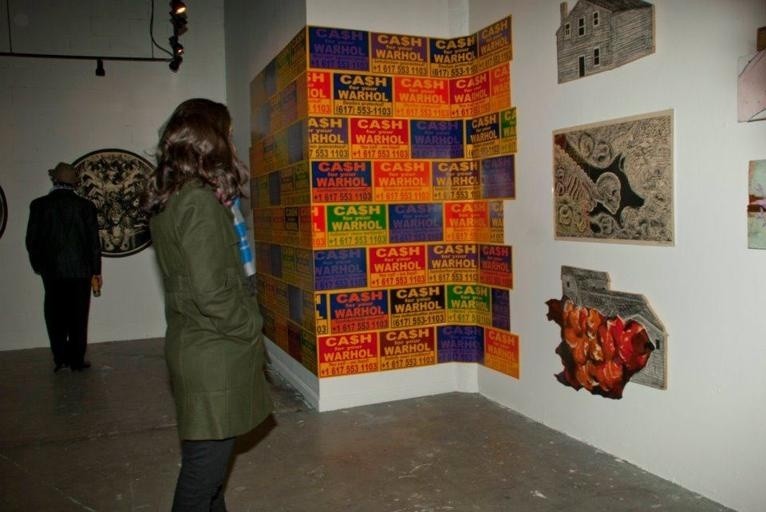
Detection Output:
[54,362,70,374]
[71,360,92,371]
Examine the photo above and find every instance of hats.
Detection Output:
[48,162,80,185]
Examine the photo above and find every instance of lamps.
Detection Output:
[166,0,189,73]
[95,57,105,77]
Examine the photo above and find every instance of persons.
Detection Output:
[26,162,103,374]
[140,96,274,510]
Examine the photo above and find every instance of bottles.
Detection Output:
[92,274,101,297]
